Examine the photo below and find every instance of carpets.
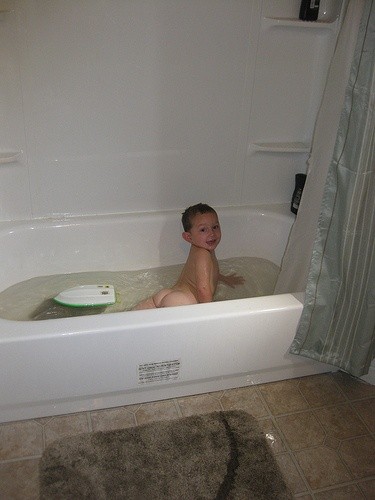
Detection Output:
[40,411,294,500]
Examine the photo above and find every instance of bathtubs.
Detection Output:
[0,204,336,423]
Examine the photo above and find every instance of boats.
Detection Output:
[53,285,115,308]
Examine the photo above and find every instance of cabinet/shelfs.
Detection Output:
[250,17,332,154]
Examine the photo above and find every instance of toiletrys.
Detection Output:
[299,0,320,21]
[290,173,307,215]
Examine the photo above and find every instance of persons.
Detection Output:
[129,203,246,310]
